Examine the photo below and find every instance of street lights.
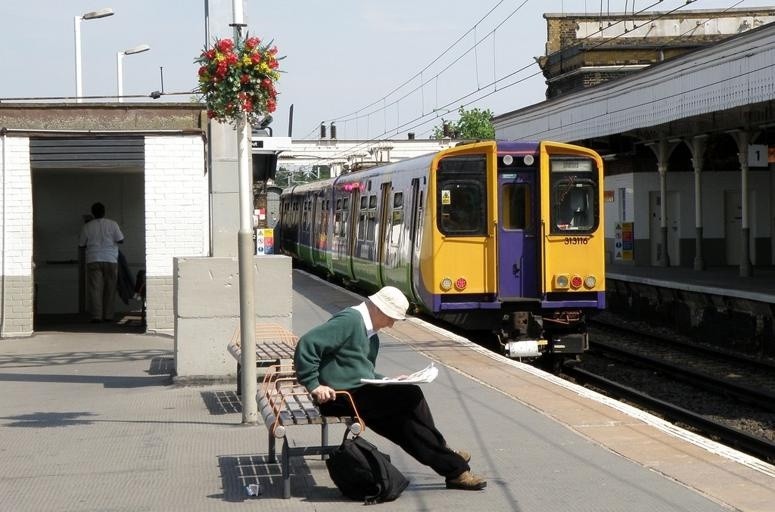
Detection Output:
[117,44,150,103]
[74,7,114,103]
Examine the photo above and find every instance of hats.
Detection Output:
[368,285,410,323]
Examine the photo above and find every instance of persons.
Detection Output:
[78,201,124,323]
[294,286,488,490]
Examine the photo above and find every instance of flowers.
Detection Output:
[190,30,290,132]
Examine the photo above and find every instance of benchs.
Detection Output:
[225,322,366,500]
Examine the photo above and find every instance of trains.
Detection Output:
[266,139,607,372]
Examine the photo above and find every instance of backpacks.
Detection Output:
[323,436,410,507]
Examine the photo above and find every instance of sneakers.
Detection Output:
[445,443,471,462]
[444,470,487,490]
